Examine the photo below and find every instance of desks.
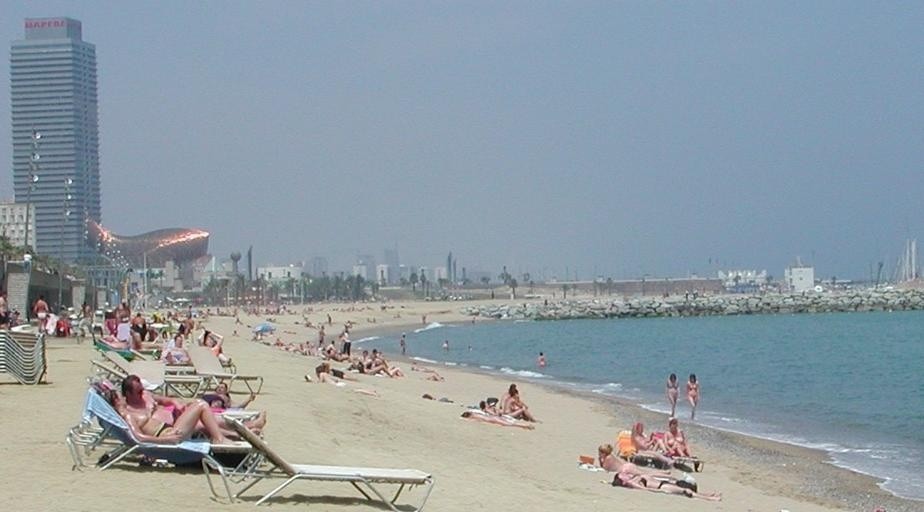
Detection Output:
[149,323,168,344]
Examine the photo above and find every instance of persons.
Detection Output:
[599,419,723,502]
[687,373,701,419]
[538,352,545,365]
[667,374,681,418]
[460,383,543,429]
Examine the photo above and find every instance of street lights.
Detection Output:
[23,129,42,246]
[58,170,73,314]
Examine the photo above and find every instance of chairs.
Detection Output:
[615,429,665,470]
[651,429,706,473]
[0,327,47,387]
[78,335,263,470]
[199,409,433,512]
